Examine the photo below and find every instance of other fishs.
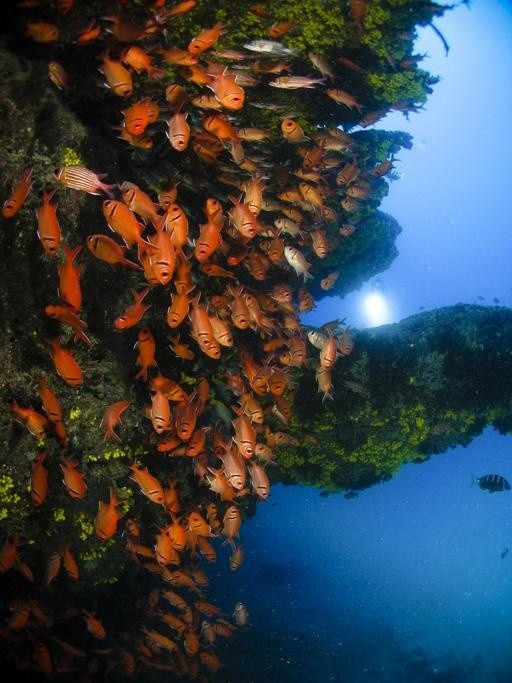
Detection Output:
[0,0,401,683]
